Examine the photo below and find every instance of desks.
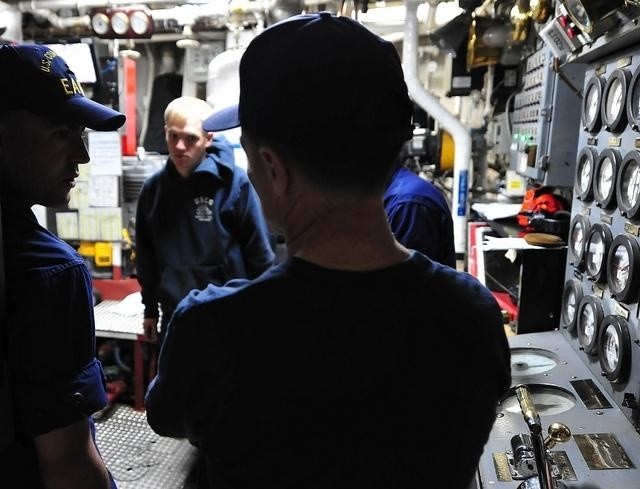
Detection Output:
[94,299,163,411]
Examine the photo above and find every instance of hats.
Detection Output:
[0,44,126,133]
[201,11,411,133]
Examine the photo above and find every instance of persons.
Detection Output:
[145,16,511,489]
[1,44,124,489]
[382,150,454,275]
[131,97,276,365]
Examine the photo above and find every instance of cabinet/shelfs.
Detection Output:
[468,199,567,335]
